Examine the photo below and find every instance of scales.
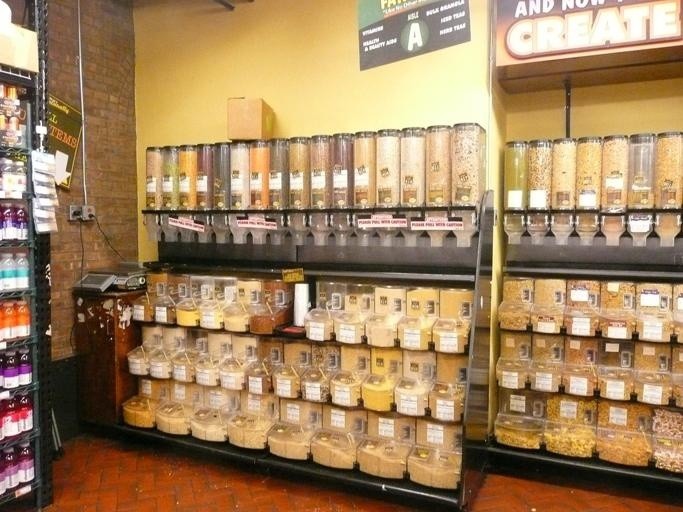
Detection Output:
[72,262,147,292]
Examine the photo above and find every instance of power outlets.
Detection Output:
[82,206,96,221]
[70,205,81,220]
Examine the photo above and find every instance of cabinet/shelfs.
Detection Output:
[75,288,145,426]
[490,0,683,510]
[1,0,53,508]
[115,190,494,511]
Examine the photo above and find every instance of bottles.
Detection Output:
[0,157,35,498]
[144,122,485,248]
[502,132,683,247]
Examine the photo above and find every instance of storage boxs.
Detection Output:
[227,98,265,140]
[1,24,40,74]
